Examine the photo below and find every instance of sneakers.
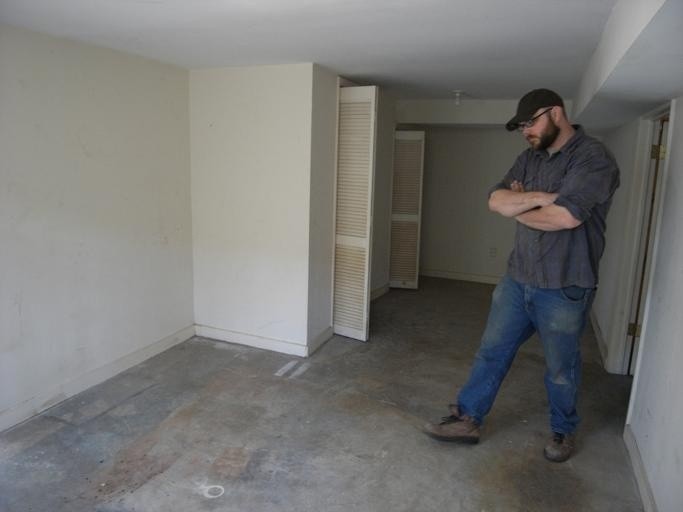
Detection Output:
[417,403,481,443]
[544,432,569,462]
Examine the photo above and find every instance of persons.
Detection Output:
[419,89,620,463]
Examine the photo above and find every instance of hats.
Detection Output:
[506,88,563,131]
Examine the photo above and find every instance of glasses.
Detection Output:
[516,108,552,132]
